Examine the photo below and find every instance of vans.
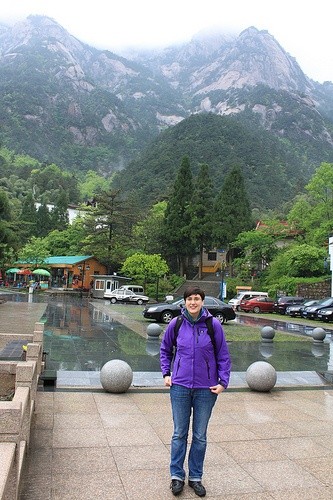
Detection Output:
[228,292,268,312]
[120,285,144,295]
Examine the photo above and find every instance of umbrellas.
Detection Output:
[5,268,50,282]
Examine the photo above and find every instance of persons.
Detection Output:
[323,257,330,275]
[222,260,227,273]
[89,279,93,289]
[159,285,231,496]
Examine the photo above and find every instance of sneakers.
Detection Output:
[170,479,185,496]
[188,480,206,497]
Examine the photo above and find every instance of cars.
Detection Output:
[143,294,236,324]
[303,297,333,320]
[239,296,275,313]
[286,299,319,319]
[103,289,150,305]
[317,308,333,323]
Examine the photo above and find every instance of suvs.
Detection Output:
[274,297,304,315]
